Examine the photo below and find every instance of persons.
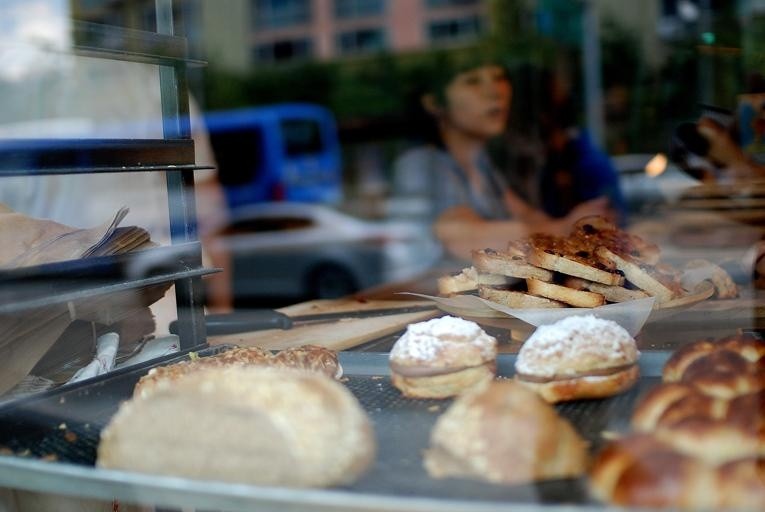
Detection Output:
[382,41,617,283]
[538,120,630,227]
[185,87,238,315]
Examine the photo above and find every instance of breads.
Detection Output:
[95,214,765,512]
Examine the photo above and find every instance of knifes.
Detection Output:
[169,304,436,337]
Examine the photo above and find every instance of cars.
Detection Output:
[130,203,434,301]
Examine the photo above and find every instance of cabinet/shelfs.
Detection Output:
[0,0,224,423]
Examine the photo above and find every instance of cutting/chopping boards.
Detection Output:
[208,298,445,351]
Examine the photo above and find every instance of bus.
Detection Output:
[104,105,343,238]
